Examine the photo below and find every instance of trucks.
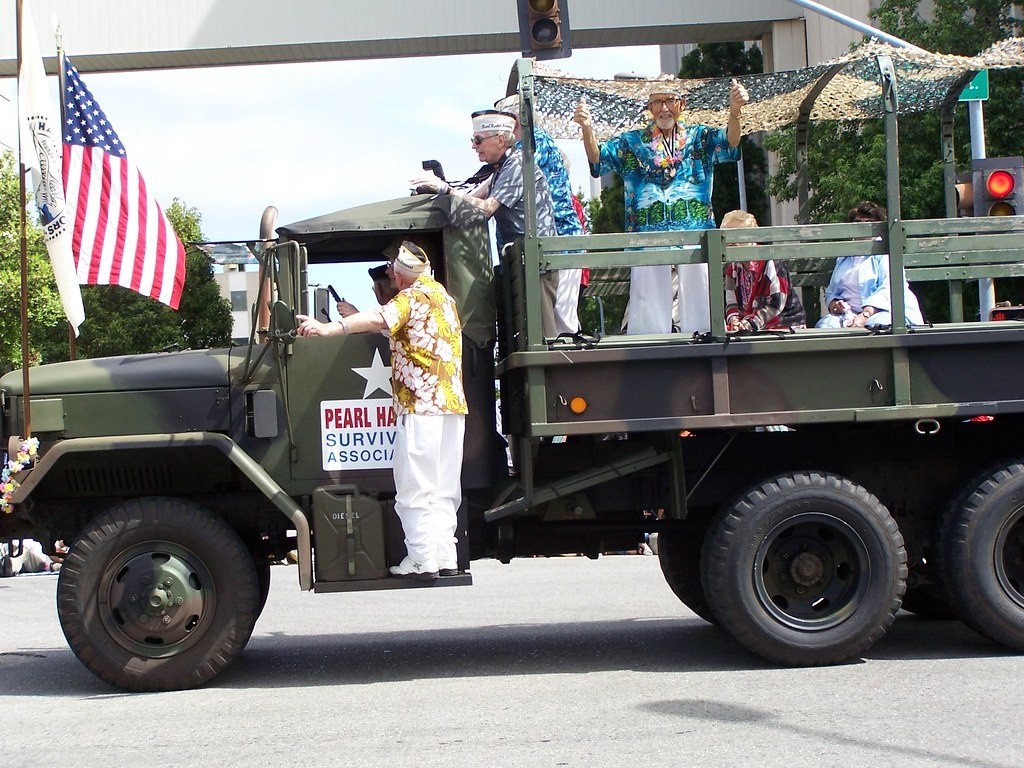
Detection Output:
[0,53,1024,695]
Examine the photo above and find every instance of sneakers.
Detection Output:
[388,562,440,581]
[439,560,458,576]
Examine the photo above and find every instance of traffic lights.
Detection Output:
[517,0,573,62]
[971,155,1024,234]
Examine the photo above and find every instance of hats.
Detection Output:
[494,93,520,113]
[471,110,517,133]
[382,243,401,258]
[650,91,683,102]
[394,240,430,274]
[368,264,390,281]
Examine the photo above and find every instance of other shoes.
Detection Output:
[3,555,13,577]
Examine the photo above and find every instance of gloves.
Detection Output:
[409,175,449,195]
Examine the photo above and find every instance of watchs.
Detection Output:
[862,311,870,318]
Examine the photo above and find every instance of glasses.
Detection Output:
[651,98,681,106]
[471,134,499,145]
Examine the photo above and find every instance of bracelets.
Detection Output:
[338,318,349,336]
[729,109,742,120]
[446,186,452,195]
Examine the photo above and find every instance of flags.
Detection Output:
[61,51,185,311]
[13,0,86,338]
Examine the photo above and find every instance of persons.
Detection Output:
[816,201,924,329]
[406,93,592,341]
[573,76,750,334]
[293,241,470,581]
[719,209,808,332]
